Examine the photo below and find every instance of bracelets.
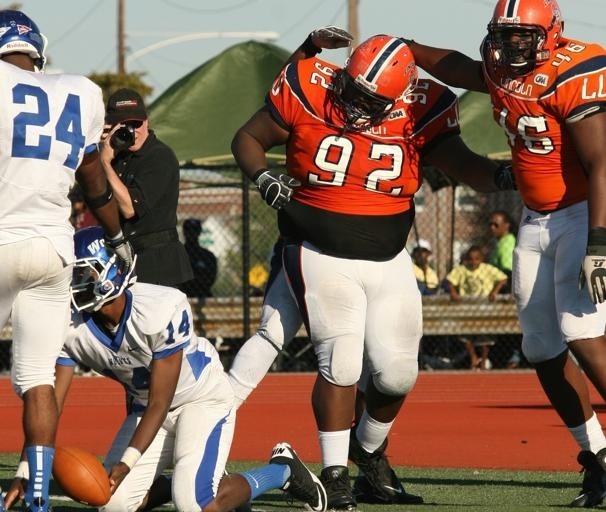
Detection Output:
[586,226,606,247]
[119,445,141,471]
[13,459,32,481]
[297,36,323,58]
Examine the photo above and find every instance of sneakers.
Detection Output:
[570,447,606,507]
[270,426,423,512]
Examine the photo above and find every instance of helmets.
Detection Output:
[487,0,564,75]
[70,226,137,313]
[0,10,48,58]
[331,34,417,125]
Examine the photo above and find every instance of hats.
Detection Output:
[108,88,147,123]
[414,239,432,252]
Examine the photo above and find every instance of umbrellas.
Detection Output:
[144,39,297,341]
[419,73,520,199]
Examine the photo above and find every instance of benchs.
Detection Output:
[189,293,521,369]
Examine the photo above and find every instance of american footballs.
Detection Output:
[53,446,110,505]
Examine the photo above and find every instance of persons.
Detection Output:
[0,4,142,511]
[388,1,606,511]
[4,224,330,511]
[445,244,509,370]
[67,85,195,420]
[181,217,219,299]
[411,237,444,297]
[230,32,519,512]
[226,25,364,421]
[479,208,517,369]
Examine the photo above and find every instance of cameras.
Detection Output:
[111,127,135,148]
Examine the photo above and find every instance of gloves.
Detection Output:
[311,27,352,48]
[109,230,136,274]
[577,227,606,304]
[254,168,301,210]
